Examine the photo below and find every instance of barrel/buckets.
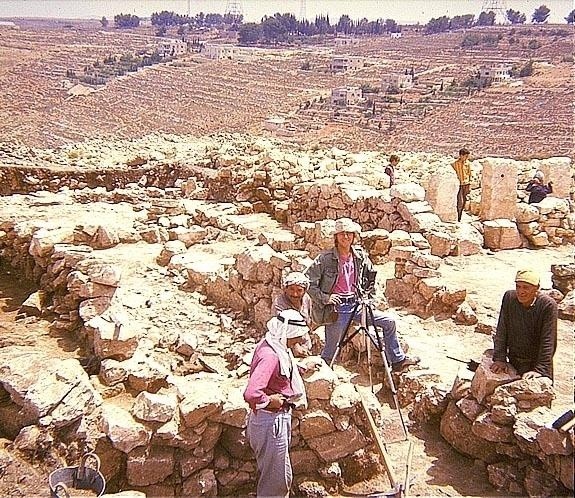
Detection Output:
[49,454,106,498]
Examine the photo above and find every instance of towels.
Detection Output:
[264,331,311,414]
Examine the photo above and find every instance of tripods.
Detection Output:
[330,300,409,441]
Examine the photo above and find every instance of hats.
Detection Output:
[514,268,541,287]
[283,272,311,290]
[266,308,310,339]
[329,217,363,235]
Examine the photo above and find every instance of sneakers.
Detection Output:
[392,356,422,372]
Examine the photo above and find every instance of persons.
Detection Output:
[384,154,400,187]
[303,217,421,372]
[524,170,552,203]
[270,272,313,358]
[488,268,559,388]
[450,147,474,222]
[243,308,311,498]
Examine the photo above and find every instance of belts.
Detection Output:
[340,296,356,303]
